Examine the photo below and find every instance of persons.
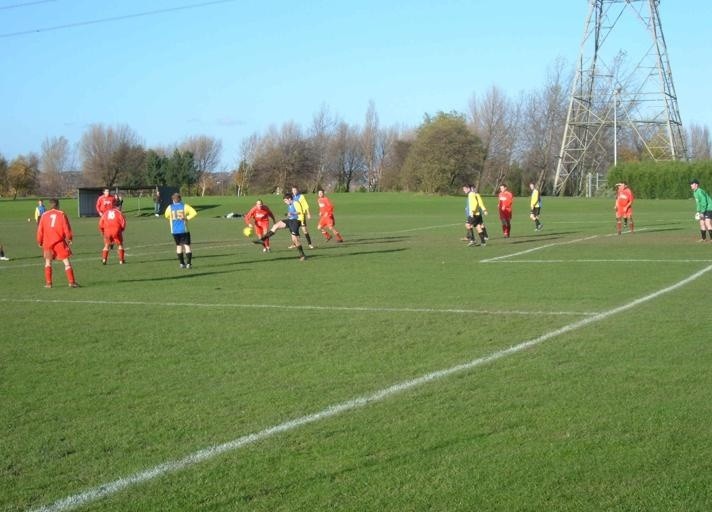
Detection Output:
[153,191,163,217]
[165,193,198,269]
[462,182,543,248]
[95,188,127,265]
[689,179,712,242]
[316,189,342,243]
[1,246,10,261]
[614,181,634,235]
[251,188,313,262]
[35,198,79,288]
[245,199,276,252]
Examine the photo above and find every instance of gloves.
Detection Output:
[695,212,700,221]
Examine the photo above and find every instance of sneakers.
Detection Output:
[504,232,510,239]
[298,256,307,262]
[462,234,491,247]
[322,236,333,243]
[120,260,127,264]
[252,238,264,246]
[179,264,193,269]
[0,256,10,261]
[533,224,544,232]
[262,245,271,254]
[102,260,107,265]
[695,238,712,243]
[288,244,299,248]
[68,282,82,288]
[336,239,344,244]
[43,283,53,288]
[308,244,315,249]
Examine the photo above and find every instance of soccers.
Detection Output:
[242,225,252,236]
